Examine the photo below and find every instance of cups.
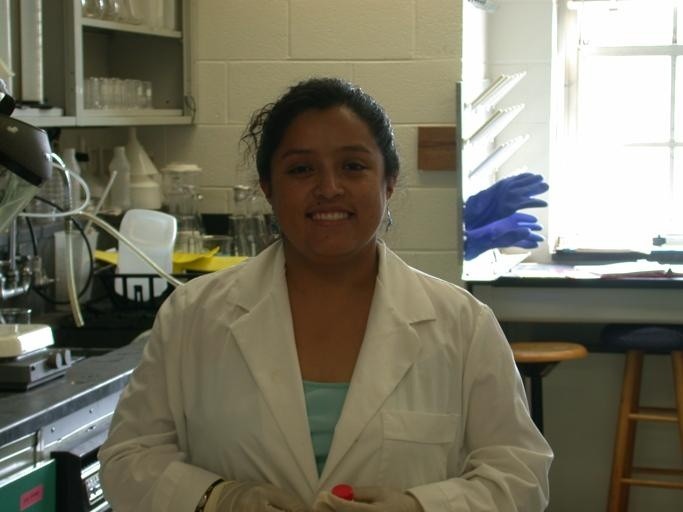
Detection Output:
[84,77,152,109]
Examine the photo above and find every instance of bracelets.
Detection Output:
[190,475,226,511]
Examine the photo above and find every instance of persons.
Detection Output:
[94,75,557,512]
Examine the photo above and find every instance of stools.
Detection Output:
[508,341,589,436]
[596,322,683,512]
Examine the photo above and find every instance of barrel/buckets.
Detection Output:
[112,206,178,304]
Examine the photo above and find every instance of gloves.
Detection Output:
[463,212,544,261]
[464,172,550,232]
[310,485,423,512]
[204,479,310,512]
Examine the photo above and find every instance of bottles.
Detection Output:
[107,146,130,210]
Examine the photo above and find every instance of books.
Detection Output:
[569,259,682,279]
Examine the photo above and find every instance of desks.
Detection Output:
[465,263,682,326]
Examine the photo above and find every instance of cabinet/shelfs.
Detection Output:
[40,0,193,128]
